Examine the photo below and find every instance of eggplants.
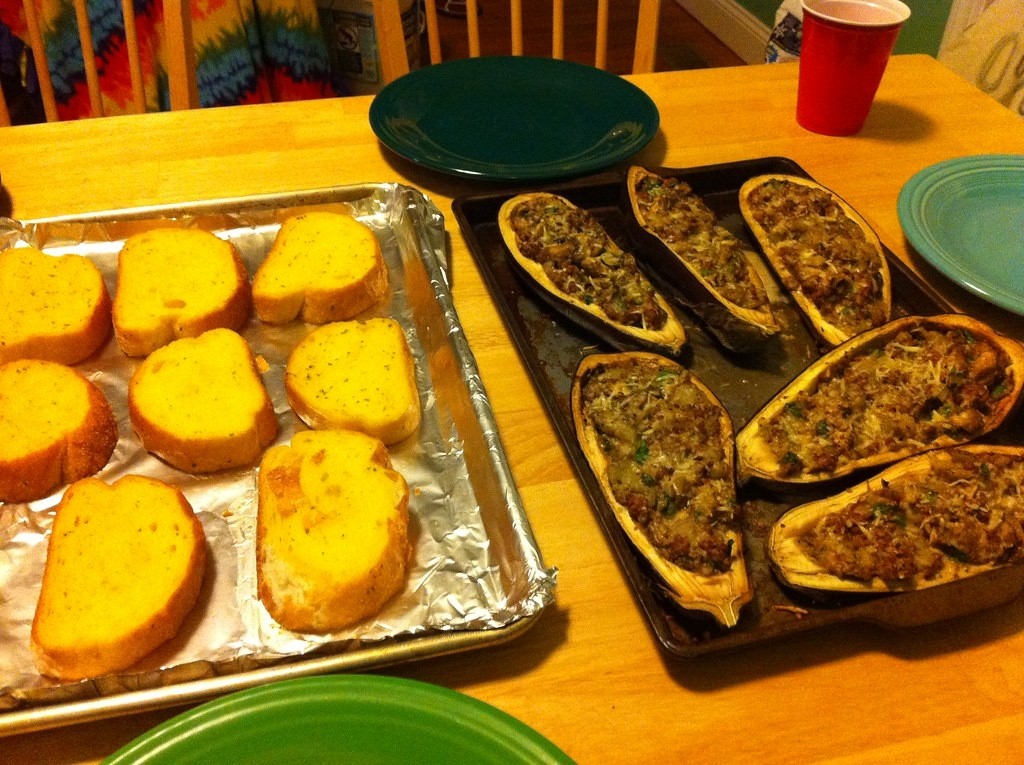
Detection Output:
[498,166,1024,629]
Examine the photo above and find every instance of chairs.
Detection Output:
[0,0,200,130]
[371,0,660,86]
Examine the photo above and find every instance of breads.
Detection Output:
[0,211,423,678]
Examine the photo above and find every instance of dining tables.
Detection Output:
[0,55,1024,765]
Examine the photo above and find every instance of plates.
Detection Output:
[97,675,576,765]
[897,152,1024,319]
[369,55,661,183]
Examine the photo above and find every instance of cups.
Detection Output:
[795,0,912,138]
